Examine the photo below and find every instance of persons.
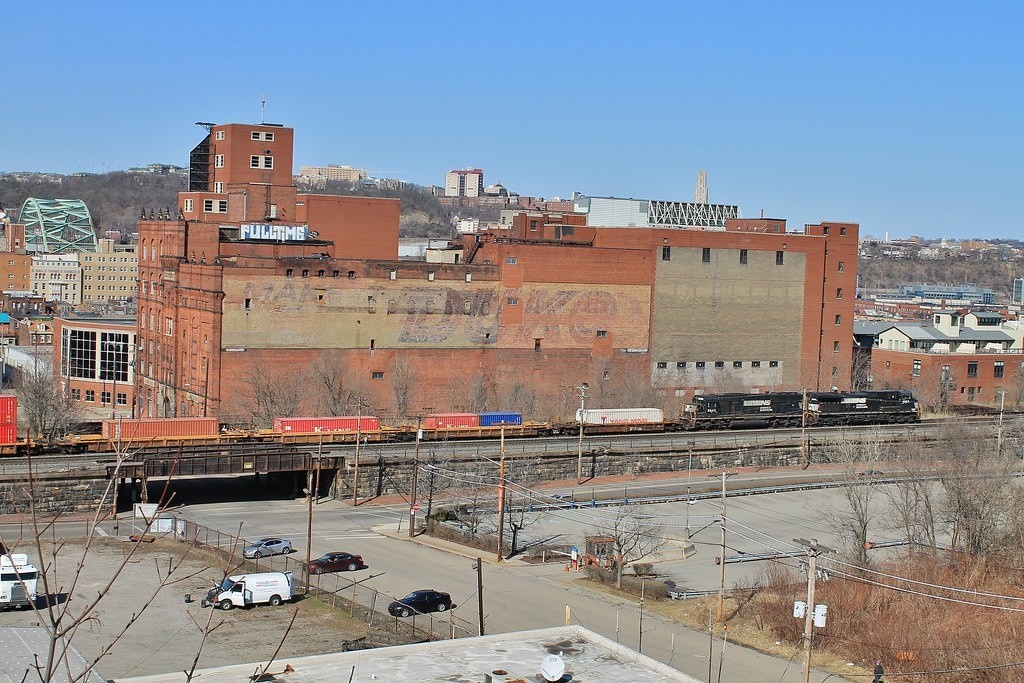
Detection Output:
[872,660,885,683]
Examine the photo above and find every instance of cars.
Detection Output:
[242,537,292,559]
[302,551,364,575]
[388,588,452,618]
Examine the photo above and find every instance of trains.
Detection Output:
[0,389,921,452]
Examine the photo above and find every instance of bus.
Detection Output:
[0,554,40,612]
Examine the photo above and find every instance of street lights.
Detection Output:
[634,511,725,621]
[997,390,1005,457]
[1009,276,1024,303]
[573,384,585,486]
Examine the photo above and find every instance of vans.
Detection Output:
[204,571,296,611]
